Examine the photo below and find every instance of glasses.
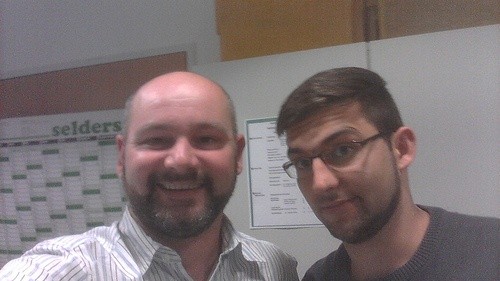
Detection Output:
[283,129,395,179]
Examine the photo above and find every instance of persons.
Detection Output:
[275,66,500,281]
[0,71,300,281]
